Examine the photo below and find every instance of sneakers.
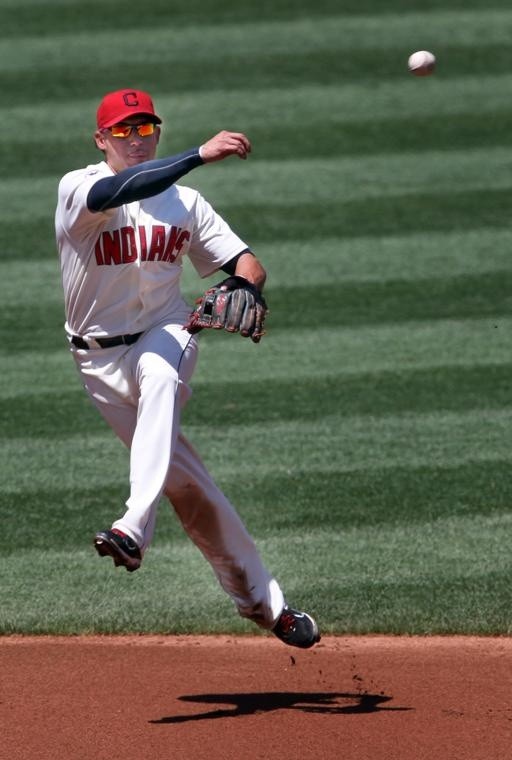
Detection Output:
[94,529,142,573]
[272,604,321,650]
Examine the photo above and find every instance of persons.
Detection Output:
[53,89,320,648]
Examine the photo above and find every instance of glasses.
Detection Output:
[108,121,158,140]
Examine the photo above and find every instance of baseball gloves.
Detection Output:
[187,275,266,342]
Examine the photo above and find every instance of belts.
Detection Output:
[68,332,139,350]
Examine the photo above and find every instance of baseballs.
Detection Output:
[408,50,436,75]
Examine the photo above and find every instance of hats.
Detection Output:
[96,89,163,129]
